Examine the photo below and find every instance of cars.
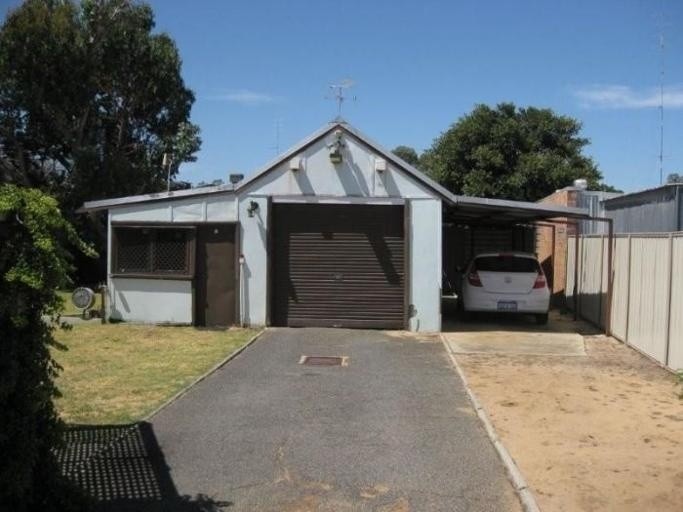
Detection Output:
[459,249,553,326]
